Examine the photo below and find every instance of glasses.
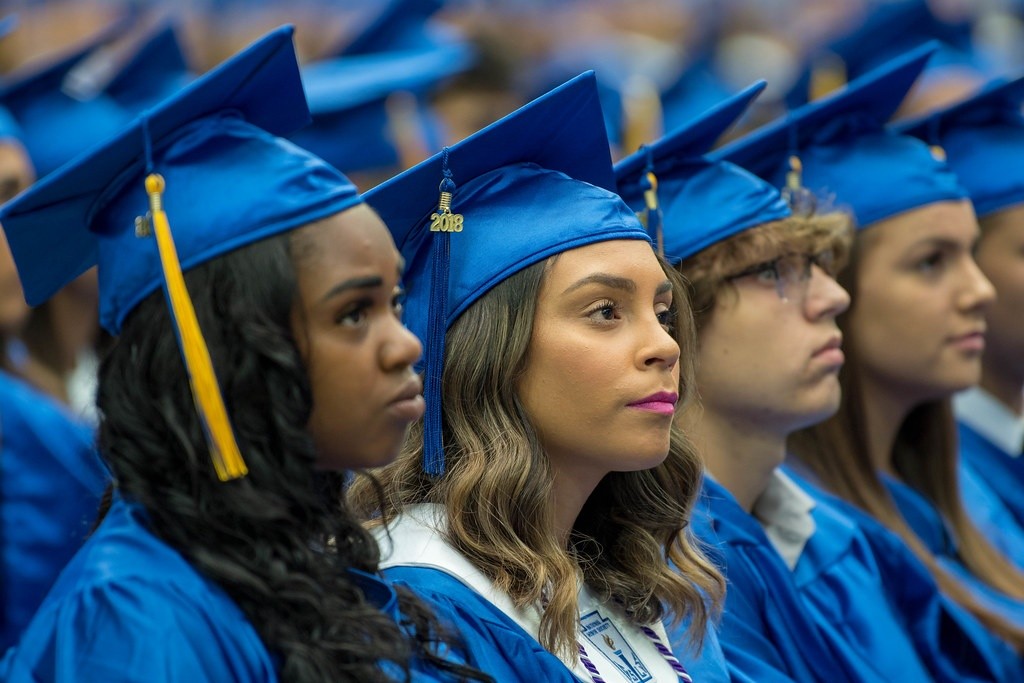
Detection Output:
[714,245,844,299]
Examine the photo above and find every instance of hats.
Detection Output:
[0,24,370,481]
[103,24,200,116]
[524,48,625,149]
[0,105,38,180]
[659,58,738,134]
[0,19,135,178]
[702,40,969,228]
[359,68,653,473]
[612,79,793,265]
[883,75,1024,215]
[337,0,442,55]
[300,43,469,174]
[827,0,974,83]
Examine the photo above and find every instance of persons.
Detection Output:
[0,0,1024,683]
